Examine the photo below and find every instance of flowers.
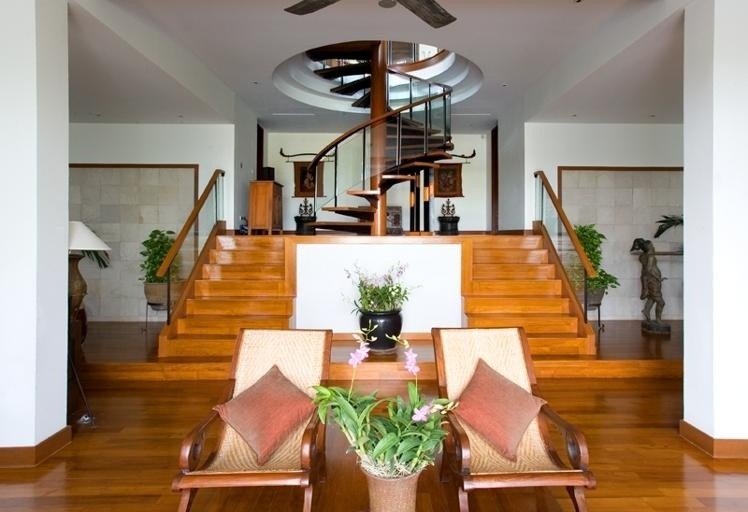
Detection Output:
[334,260,424,313]
[309,321,460,478]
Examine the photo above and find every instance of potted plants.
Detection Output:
[562,224,620,311]
[137,229,188,304]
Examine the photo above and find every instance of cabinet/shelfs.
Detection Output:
[247,181,284,235]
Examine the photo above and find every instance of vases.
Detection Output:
[356,457,429,512]
[438,217,460,234]
[358,307,403,350]
[294,216,316,235]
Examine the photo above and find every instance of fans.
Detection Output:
[284,1,459,30]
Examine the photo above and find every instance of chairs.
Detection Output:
[429,325,599,511]
[170,326,337,511]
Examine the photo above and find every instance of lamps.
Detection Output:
[68,221,112,315]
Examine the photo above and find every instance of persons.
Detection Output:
[630,238,665,324]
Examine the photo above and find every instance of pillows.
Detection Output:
[448,357,550,463]
[212,364,320,468]
